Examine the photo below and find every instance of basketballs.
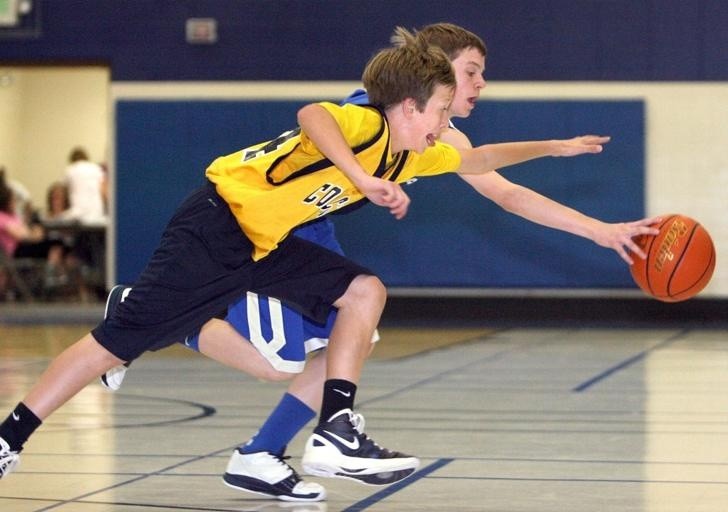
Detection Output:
[629,215,715,303]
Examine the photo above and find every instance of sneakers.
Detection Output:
[223,447,325,502]
[302,408,419,487]
[0,436,18,478]
[100,285,134,390]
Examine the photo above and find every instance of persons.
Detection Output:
[0,24,611,488]
[93,20,665,505]
[0,146,109,305]
